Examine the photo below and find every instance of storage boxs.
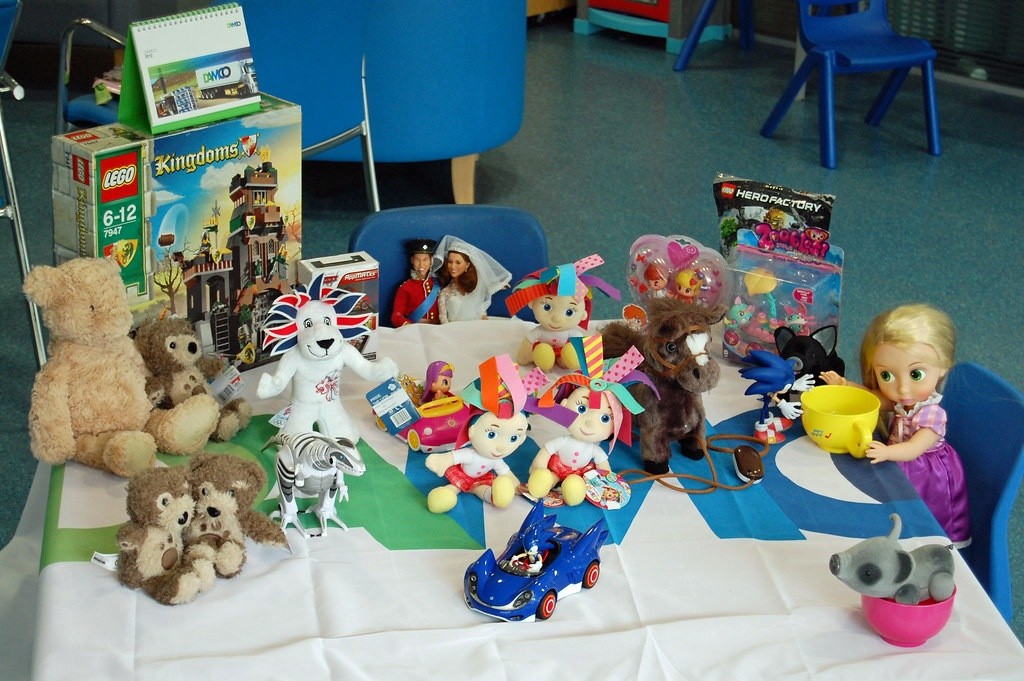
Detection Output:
[299,248,380,329]
[51,91,304,320]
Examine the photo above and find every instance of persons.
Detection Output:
[390,238,442,327]
[432,234,512,323]
[643,264,673,299]
[675,270,702,305]
[819,305,972,551]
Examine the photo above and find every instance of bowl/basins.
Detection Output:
[861,585,960,648]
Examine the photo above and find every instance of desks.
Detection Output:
[1,318,1024,679]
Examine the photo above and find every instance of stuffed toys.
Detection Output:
[24,253,720,603]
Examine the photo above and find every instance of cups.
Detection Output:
[799,384,881,459]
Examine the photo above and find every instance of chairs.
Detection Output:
[930,361,1023,635]
[211,0,529,204]
[355,202,545,327]
[760,0,942,168]
